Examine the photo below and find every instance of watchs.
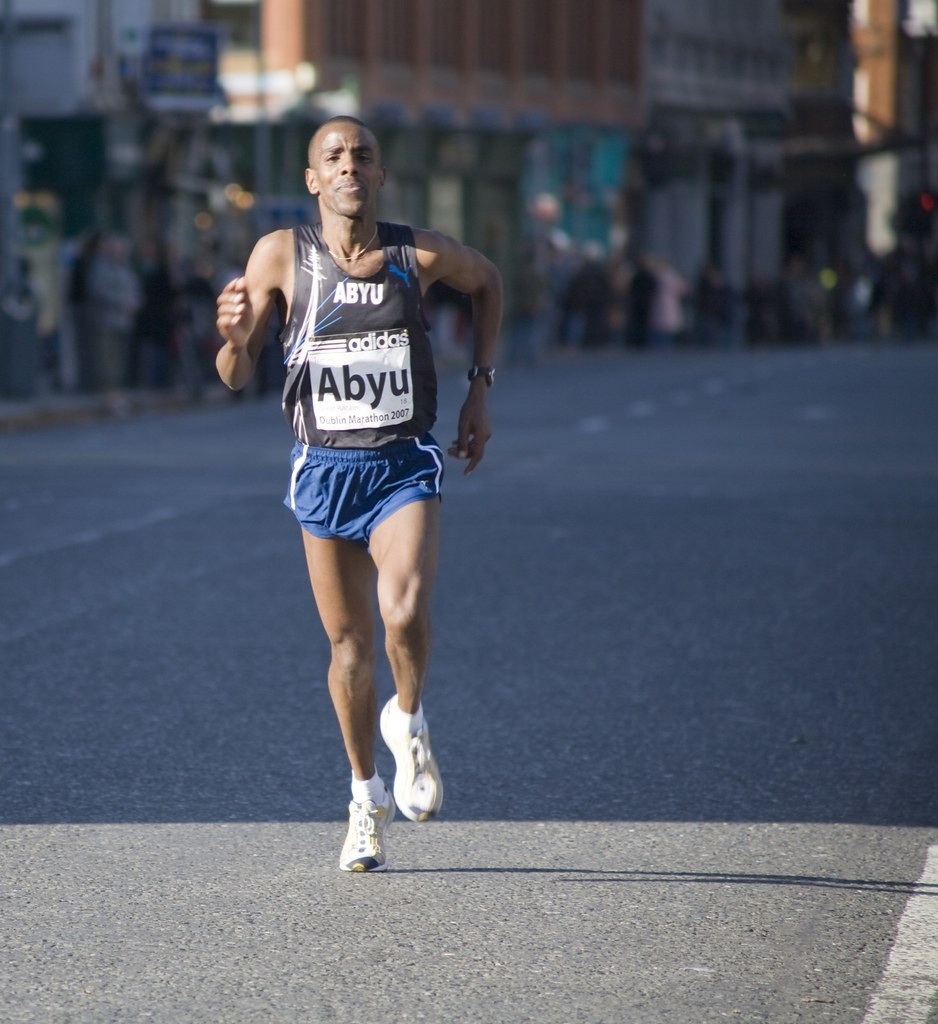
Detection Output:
[467,367,495,386]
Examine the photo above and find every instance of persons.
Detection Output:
[534,180,659,344]
[17,223,286,400]
[216,115,503,874]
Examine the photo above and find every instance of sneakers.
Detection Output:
[379,694,442,822]
[339,783,395,872]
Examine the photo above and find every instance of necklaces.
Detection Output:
[330,226,378,260]
